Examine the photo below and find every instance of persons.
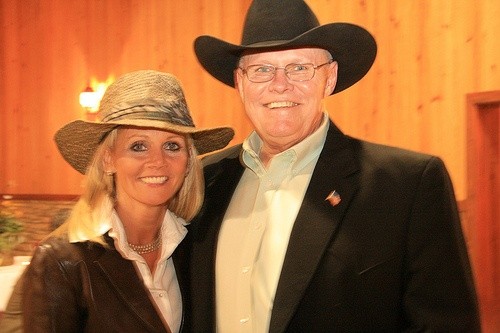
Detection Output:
[192,0,485,333]
[23,70,235,333]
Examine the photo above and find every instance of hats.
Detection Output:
[54,71,236,177]
[194,0,377,95]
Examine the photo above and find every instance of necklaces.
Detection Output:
[128,234,160,254]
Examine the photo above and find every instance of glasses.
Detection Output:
[237,58,335,82]
